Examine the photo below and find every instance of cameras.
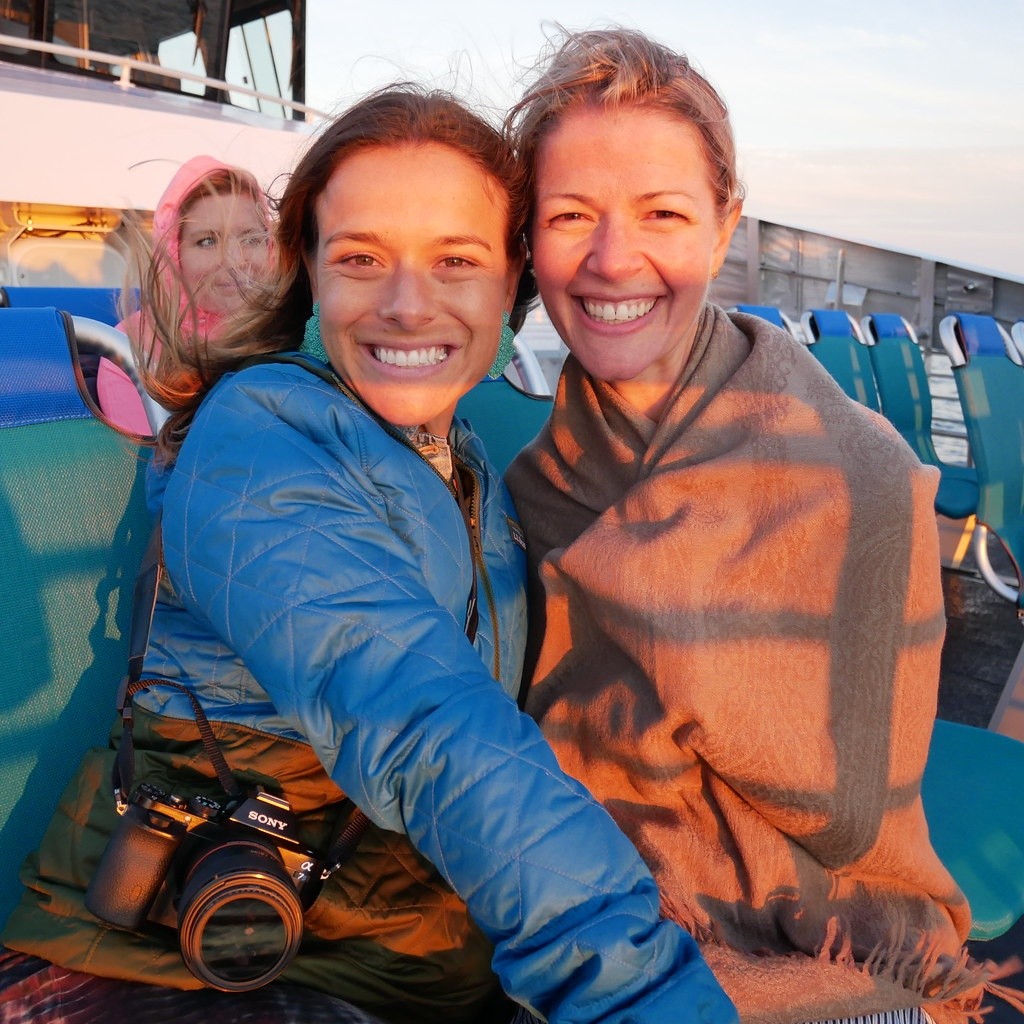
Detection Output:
[85,781,327,993]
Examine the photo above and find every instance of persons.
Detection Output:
[496,30,1024,1023]
[97,155,275,437]
[1,89,742,1023]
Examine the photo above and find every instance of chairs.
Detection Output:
[939,314,1023,624]
[799,309,879,414]
[920,719,1023,940]
[453,335,555,472]
[860,312,979,519]
[0,306,171,943]
[733,304,796,341]
